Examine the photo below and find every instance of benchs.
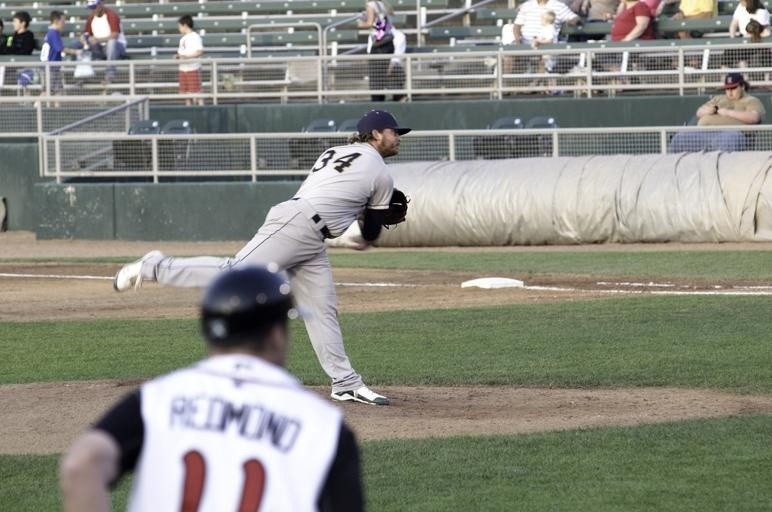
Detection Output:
[0,0,772,98]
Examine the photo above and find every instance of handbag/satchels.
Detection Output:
[17,68,40,105]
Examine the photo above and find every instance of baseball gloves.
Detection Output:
[365,188,406,226]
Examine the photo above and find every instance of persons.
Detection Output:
[177,15,205,107]
[58,266,362,512]
[498,0,772,96]
[669,73,766,153]
[357,0,393,102]
[1,0,129,110]
[386,26,407,102]
[114,110,413,405]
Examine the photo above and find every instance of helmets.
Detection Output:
[197,267,296,348]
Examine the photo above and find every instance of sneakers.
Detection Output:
[330,384,390,407]
[113,250,165,294]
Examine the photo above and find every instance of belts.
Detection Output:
[291,196,332,239]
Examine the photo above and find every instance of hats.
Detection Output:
[720,73,744,90]
[356,110,411,137]
[84,0,100,10]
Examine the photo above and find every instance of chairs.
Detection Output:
[112,113,756,170]
